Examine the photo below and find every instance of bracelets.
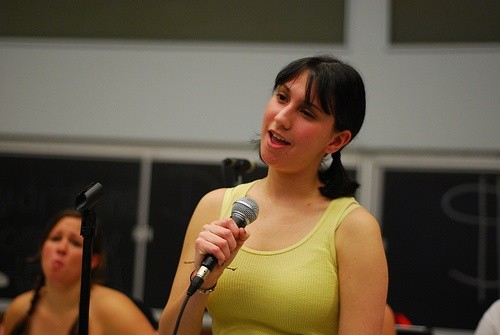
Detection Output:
[188,271,216,295]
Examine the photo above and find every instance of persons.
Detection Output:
[0,209,158,335]
[159,55,388,335]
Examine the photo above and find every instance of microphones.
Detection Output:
[224,157,257,175]
[188,196,260,294]
